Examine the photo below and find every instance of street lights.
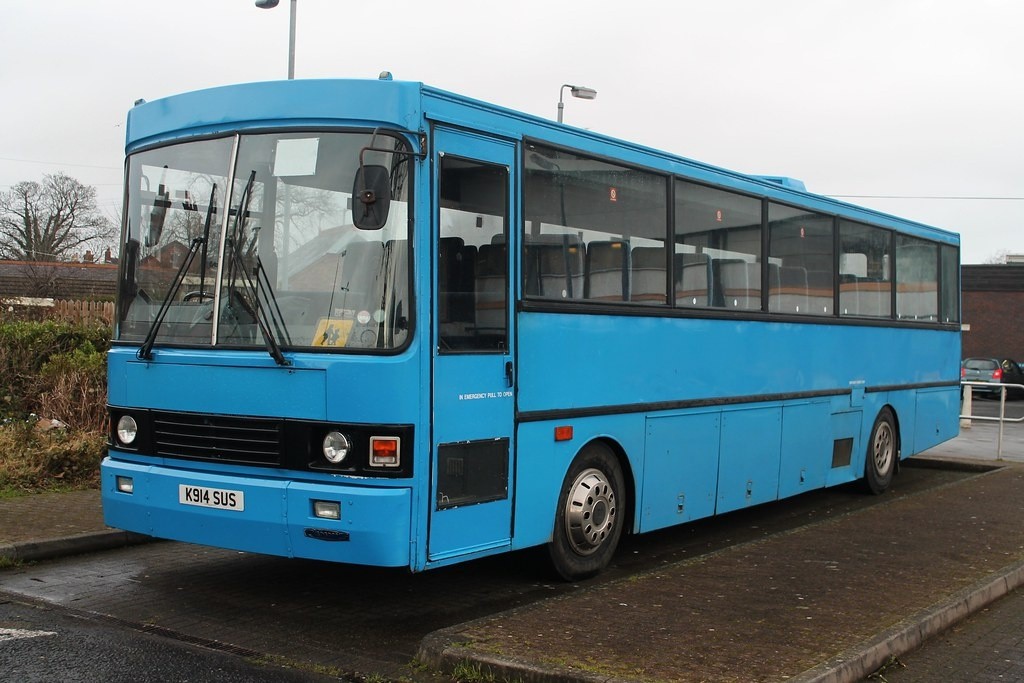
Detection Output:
[255,0,297,80]
[557,84,598,123]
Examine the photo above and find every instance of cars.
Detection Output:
[960,356,1024,401]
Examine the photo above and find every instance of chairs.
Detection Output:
[339,232,937,351]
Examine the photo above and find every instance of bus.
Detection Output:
[100,71,965,591]
